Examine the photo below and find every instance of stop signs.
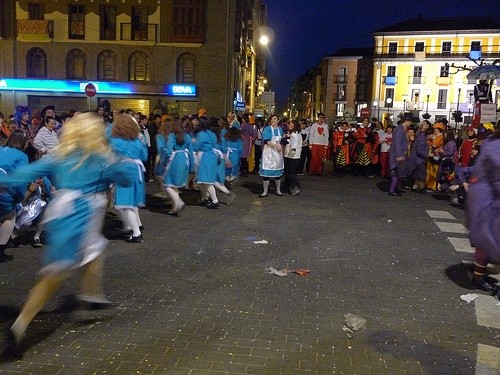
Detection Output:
[85,83,96,97]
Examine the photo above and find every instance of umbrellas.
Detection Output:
[466,64,500,79]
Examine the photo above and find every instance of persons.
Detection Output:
[474,73,492,104]
[0,99,500,375]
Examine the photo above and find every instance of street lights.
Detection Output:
[374,101,378,118]
[467,104,472,126]
[387,97,392,118]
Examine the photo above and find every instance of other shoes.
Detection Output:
[116,225,144,243]
[0,236,42,262]
[404,186,435,192]
[388,190,405,196]
[260,193,283,197]
[106,212,118,218]
[6,330,23,359]
[472,276,498,292]
[197,193,235,209]
[292,188,301,196]
[448,199,466,209]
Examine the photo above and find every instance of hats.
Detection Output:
[250,116,255,122]
[199,109,208,117]
[432,123,444,129]
[400,113,415,120]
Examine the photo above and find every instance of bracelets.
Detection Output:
[28,188,33,192]
